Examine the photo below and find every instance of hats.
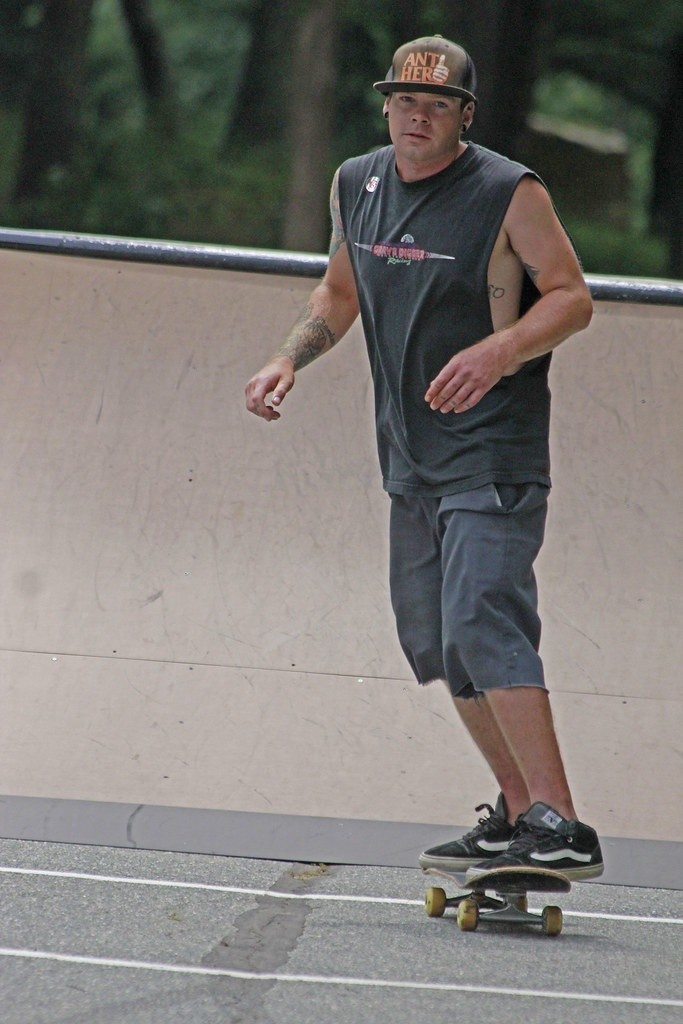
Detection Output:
[372,34,477,102]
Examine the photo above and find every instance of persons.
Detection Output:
[244,33,606,882]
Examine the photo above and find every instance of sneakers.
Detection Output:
[418,792,517,870]
[466,801,604,881]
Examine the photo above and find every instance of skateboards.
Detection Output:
[416,861,570,941]
